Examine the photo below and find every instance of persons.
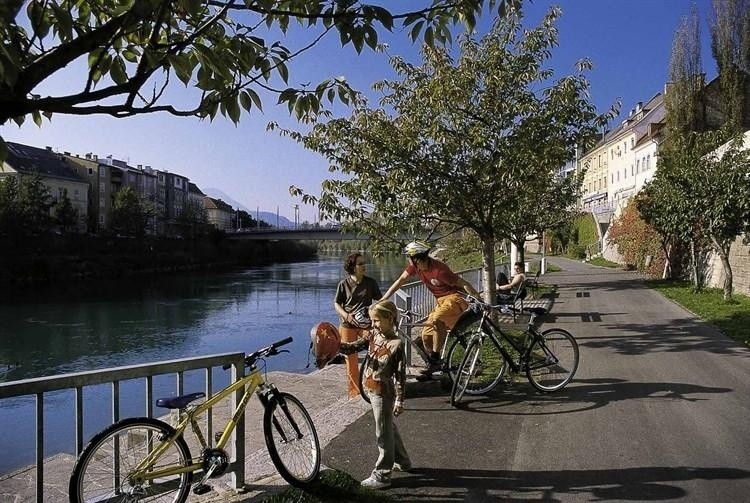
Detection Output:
[339,299,412,492]
[378,240,482,381]
[496,262,526,294]
[334,253,382,404]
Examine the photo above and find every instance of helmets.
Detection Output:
[355,305,371,328]
[400,239,432,256]
[311,320,341,369]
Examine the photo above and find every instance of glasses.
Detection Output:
[355,260,366,265]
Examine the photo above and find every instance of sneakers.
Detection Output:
[416,372,432,380]
[392,461,411,471]
[361,476,391,489]
[420,358,441,373]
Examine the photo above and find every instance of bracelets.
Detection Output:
[499,286,500,290]
[346,314,349,322]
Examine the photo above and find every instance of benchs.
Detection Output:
[477,277,530,321]
[506,269,542,300]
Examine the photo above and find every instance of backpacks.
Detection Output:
[497,272,510,293]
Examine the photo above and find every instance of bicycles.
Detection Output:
[448,290,579,405]
[356,306,507,408]
[68,336,322,503]
